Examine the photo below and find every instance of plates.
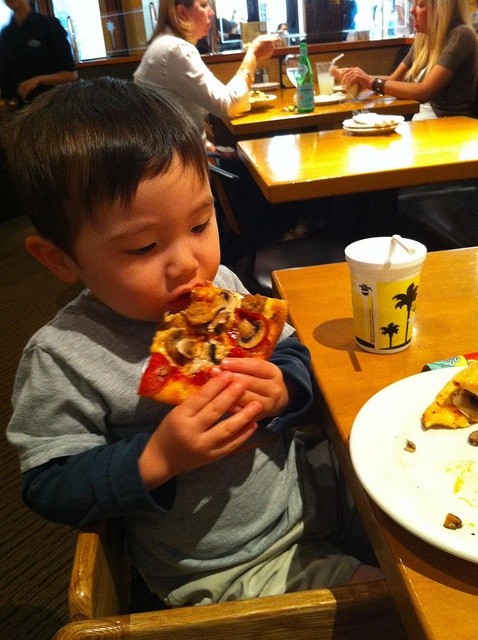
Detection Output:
[348,363,478,565]
[250,96,277,107]
[255,81,285,91]
[342,114,406,135]
[318,95,350,103]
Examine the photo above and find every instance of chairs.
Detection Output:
[399,193,478,247]
[196,117,243,241]
[52,400,411,640]
[206,151,348,295]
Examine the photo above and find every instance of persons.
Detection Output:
[2,0,79,108]
[3,75,317,610]
[278,23,289,31]
[227,19,241,35]
[338,0,478,120]
[339,0,357,41]
[394,1,412,38]
[132,0,282,154]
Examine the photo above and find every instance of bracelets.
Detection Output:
[372,78,385,95]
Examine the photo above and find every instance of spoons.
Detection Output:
[276,22,289,34]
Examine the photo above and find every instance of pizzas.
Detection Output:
[138,284,289,419]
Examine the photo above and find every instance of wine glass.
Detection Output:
[284,54,297,107]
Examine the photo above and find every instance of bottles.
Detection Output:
[295,43,315,114]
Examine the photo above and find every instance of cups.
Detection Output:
[316,60,337,95]
[342,233,428,354]
